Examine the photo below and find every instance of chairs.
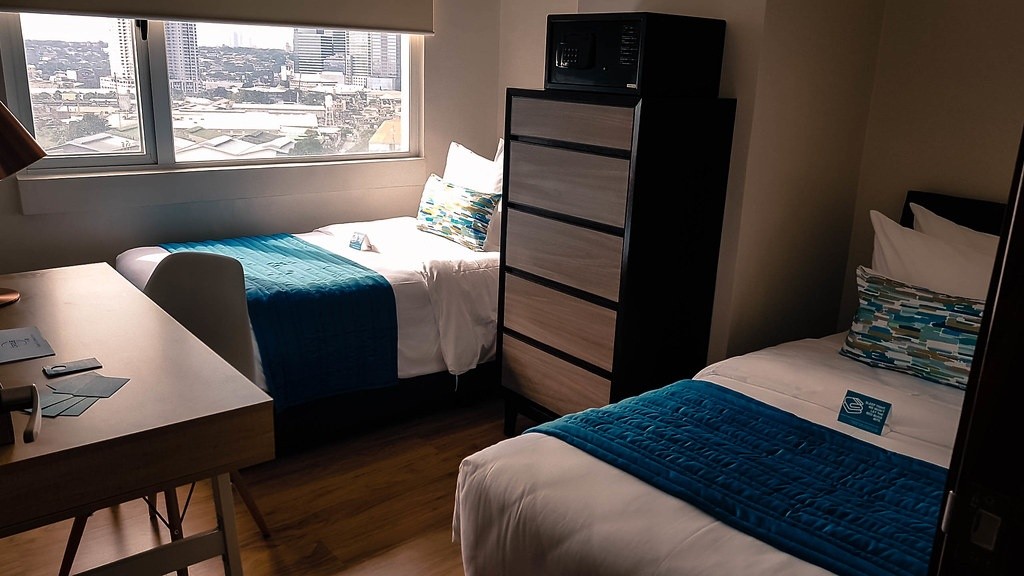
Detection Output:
[58,253,272,576]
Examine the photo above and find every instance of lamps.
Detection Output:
[0,100,47,307]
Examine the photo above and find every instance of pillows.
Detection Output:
[869,202,1000,302]
[838,267,988,389]
[416,173,501,252]
[442,138,505,246]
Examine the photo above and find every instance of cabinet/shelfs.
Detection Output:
[497,87,738,437]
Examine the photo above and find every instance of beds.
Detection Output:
[113,215,501,475]
[451,187,1009,576]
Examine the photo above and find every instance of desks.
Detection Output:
[0,262,276,576]
